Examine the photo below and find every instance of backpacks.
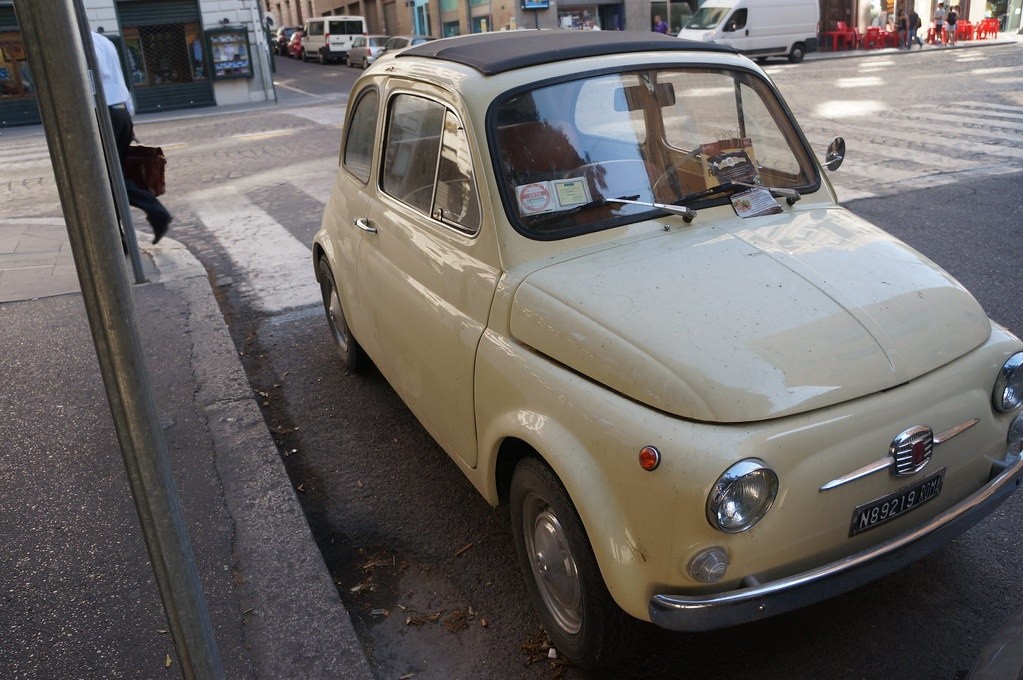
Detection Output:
[947,12,956,26]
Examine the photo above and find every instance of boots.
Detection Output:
[147,199,172,244]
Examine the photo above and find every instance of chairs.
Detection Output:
[835,17,1002,49]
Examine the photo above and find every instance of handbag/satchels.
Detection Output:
[128,137,166,197]
[912,13,921,29]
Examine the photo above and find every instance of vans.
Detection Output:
[676,0,820,63]
[376,35,437,60]
[345,36,391,69]
[299,16,369,64]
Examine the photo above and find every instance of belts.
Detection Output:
[108,104,126,109]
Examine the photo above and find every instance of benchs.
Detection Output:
[386,119,589,199]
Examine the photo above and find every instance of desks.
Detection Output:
[822,30,848,51]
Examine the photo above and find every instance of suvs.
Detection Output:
[272,26,303,56]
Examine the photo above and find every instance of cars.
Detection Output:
[287,31,304,60]
[312,28,1023,662]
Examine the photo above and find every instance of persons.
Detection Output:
[654,16,670,35]
[886,19,896,48]
[500,23,510,31]
[906,7,923,50]
[931,2,945,43]
[897,9,909,49]
[588,20,600,31]
[88,30,174,255]
[944,4,960,46]
